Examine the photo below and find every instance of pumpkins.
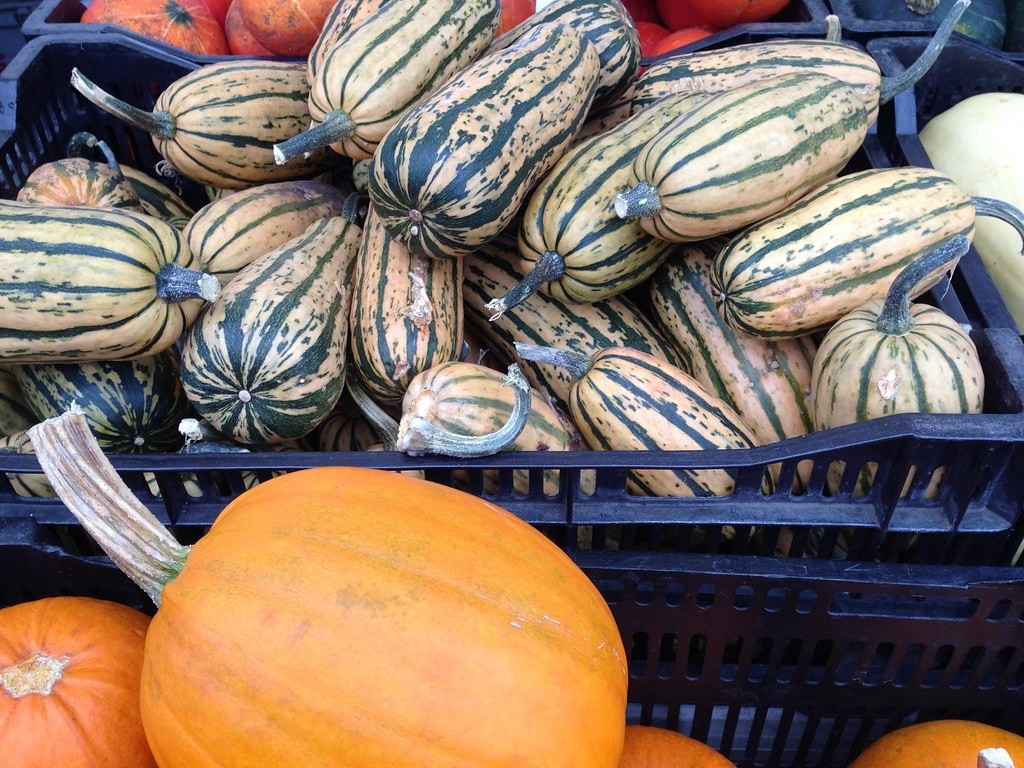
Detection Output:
[0,0,1024,768]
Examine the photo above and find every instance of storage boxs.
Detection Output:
[0,34,1024,696]
[0,514,1024,768]
[867,37,1024,340]
